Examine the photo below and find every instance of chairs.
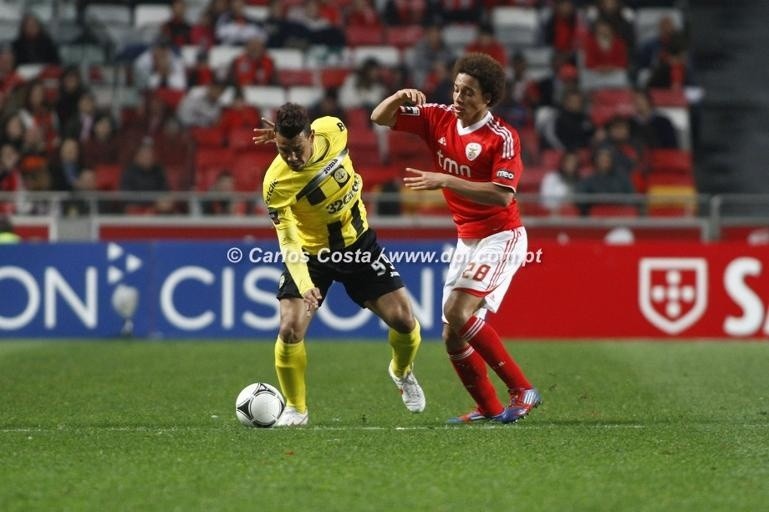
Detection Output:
[1,0,699,217]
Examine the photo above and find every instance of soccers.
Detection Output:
[236,382,285,427]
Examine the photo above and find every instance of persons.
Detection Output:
[368,52,542,425]
[249,97,429,429]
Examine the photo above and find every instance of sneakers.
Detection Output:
[272,406,308,427]
[448,386,542,424]
[388,363,426,412]
